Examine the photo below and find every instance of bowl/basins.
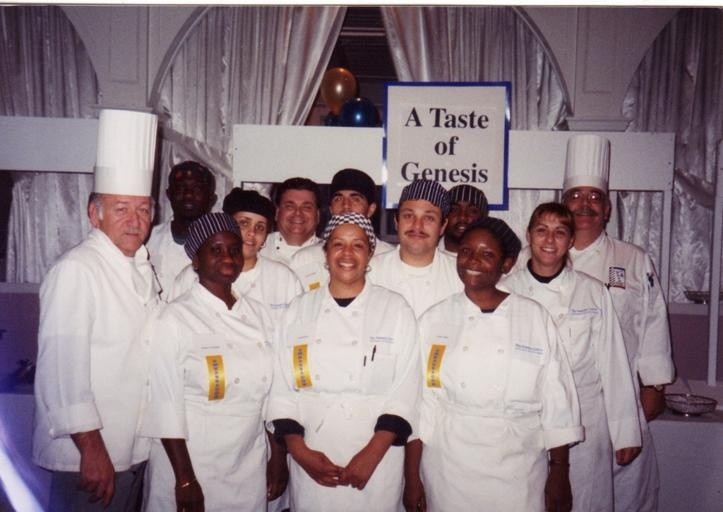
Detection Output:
[662,393,717,418]
[682,290,723,305]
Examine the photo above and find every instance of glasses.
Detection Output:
[568,190,601,202]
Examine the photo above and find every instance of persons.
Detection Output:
[501,201,642,512]
[438,184,487,256]
[403,219,587,511]
[364,181,465,321]
[222,188,301,323]
[292,170,396,294]
[34,109,163,512]
[514,133,676,512]
[260,178,321,269]
[266,212,417,512]
[138,213,288,511]
[147,161,218,299]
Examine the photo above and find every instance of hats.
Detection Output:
[323,212,376,252]
[184,214,242,260]
[222,188,276,221]
[467,217,522,257]
[329,169,377,205]
[449,184,489,217]
[399,179,451,219]
[93,108,158,196]
[563,133,611,195]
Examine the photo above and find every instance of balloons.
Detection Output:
[340,96,379,128]
[321,68,357,120]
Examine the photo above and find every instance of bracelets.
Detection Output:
[178,477,197,488]
